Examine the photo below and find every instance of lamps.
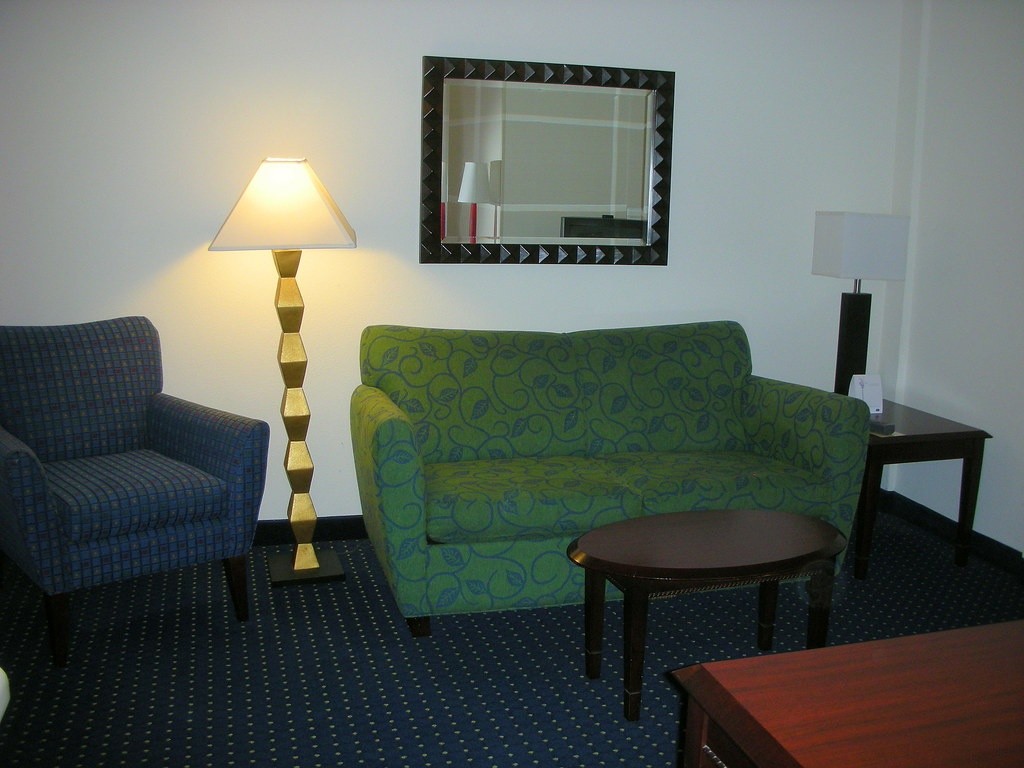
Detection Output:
[809,211,911,397]
[458,162,493,244]
[207,157,356,582]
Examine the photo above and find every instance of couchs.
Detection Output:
[0,316,271,661]
[348,320,870,638]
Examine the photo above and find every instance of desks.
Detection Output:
[851,398,993,578]
[668,615,1024,768]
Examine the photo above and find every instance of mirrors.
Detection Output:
[419,56,676,265]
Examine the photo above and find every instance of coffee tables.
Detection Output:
[566,508,848,722]
